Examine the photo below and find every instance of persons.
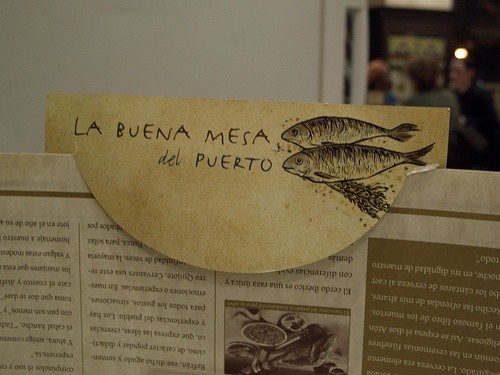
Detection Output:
[366,49,499,172]
